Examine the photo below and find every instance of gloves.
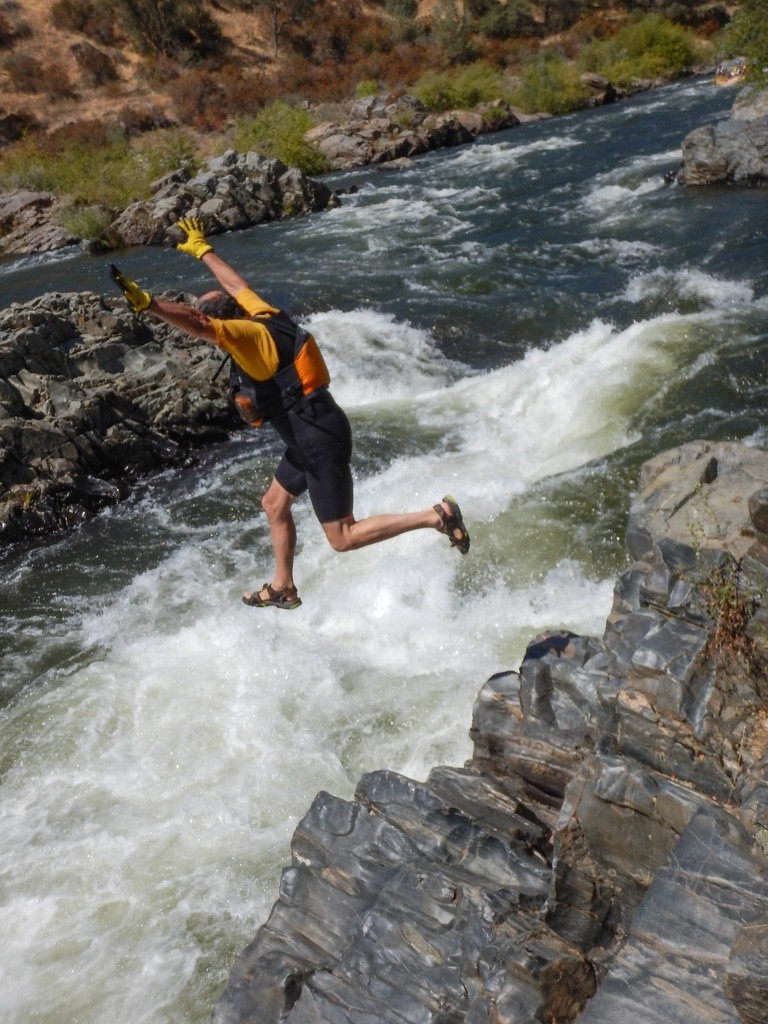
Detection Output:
[108,263,153,312]
[171,215,214,259]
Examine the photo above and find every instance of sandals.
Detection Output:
[242,583,302,609]
[433,495,470,555]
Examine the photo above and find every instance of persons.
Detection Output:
[109,213,472,610]
